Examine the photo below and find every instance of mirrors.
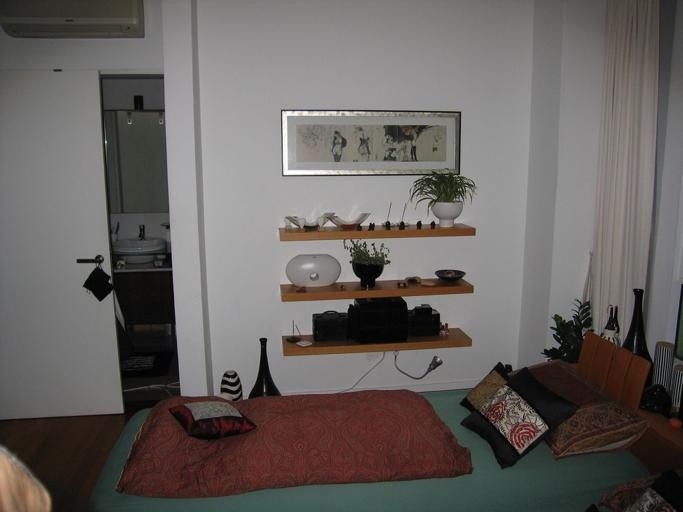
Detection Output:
[103,110,169,213]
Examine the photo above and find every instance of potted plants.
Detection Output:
[344,239,391,288]
[409,168,479,227]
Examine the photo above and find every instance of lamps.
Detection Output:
[394,352,443,380]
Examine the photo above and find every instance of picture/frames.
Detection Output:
[281,110,461,176]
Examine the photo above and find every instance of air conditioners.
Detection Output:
[0,0,145,38]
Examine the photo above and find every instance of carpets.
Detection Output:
[122,350,174,377]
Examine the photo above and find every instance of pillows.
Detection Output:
[586,473,662,512]
[459,359,649,469]
[168,401,257,439]
[625,469,683,512]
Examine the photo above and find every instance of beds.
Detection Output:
[90,388,651,512]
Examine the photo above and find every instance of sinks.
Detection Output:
[111,238,166,264]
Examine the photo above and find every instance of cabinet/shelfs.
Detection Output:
[278,223,476,356]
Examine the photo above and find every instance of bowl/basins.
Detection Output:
[435,270,466,282]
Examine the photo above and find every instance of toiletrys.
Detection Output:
[161,223,170,255]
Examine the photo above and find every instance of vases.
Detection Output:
[248,338,281,399]
[621,288,654,387]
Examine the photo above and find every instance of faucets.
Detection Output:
[139,224,145,240]
